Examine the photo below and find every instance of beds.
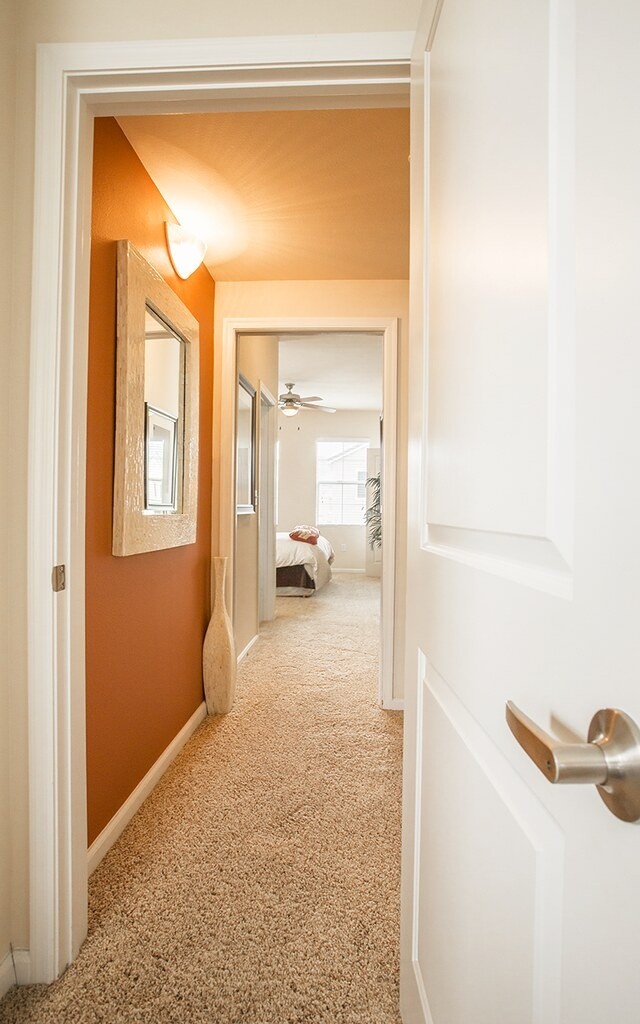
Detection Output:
[274,531,335,597]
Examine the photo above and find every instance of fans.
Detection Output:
[278,383,337,414]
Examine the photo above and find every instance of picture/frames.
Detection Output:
[235,371,256,517]
[145,402,180,514]
[112,241,199,556]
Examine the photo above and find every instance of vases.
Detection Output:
[202,555,235,713]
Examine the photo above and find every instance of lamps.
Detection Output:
[279,402,300,417]
[166,221,206,281]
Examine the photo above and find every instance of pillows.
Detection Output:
[290,525,322,545]
